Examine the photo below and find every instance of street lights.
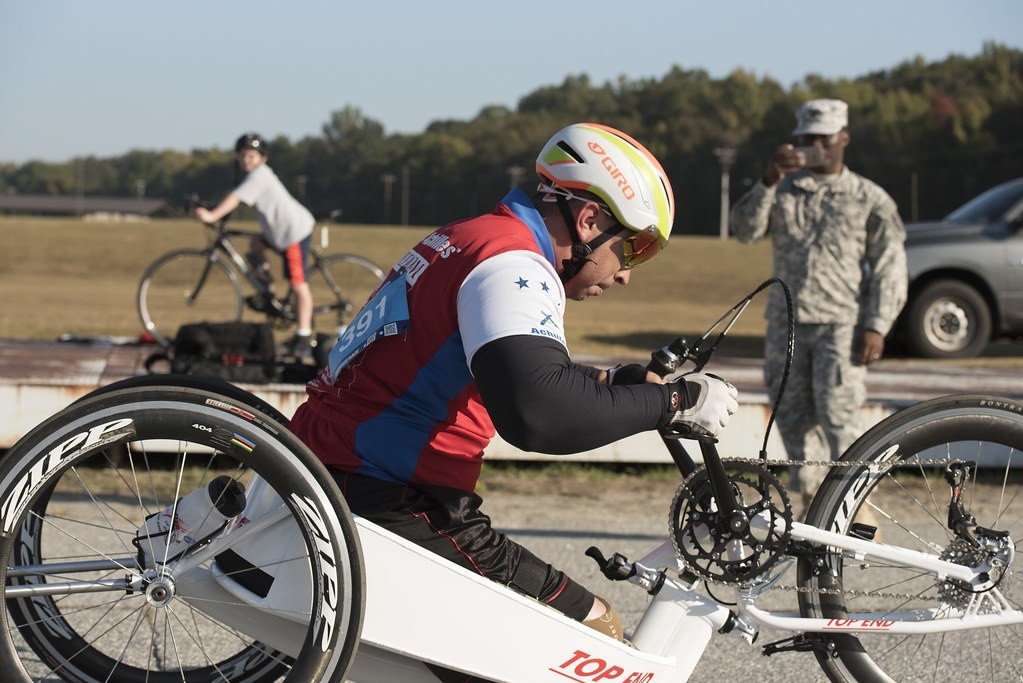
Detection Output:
[719,142,737,239]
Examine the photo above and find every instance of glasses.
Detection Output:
[620,224,668,267]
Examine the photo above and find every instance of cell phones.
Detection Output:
[795,146,825,167]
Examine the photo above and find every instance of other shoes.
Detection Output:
[279,332,313,364]
[852,507,882,542]
[241,290,275,306]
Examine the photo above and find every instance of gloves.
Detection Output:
[669,372,739,438]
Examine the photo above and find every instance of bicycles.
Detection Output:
[136,201,386,349]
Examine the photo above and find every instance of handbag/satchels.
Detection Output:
[144,322,276,385]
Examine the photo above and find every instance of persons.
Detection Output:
[200,131,315,358]
[287,122,738,648]
[730,99,908,546]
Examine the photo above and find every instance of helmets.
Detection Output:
[536,123,675,267]
[236,133,267,154]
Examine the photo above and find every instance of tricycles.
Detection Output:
[1,278,1023,683]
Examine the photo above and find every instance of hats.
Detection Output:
[792,98,849,136]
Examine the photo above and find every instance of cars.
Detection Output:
[904,178,1023,359]
